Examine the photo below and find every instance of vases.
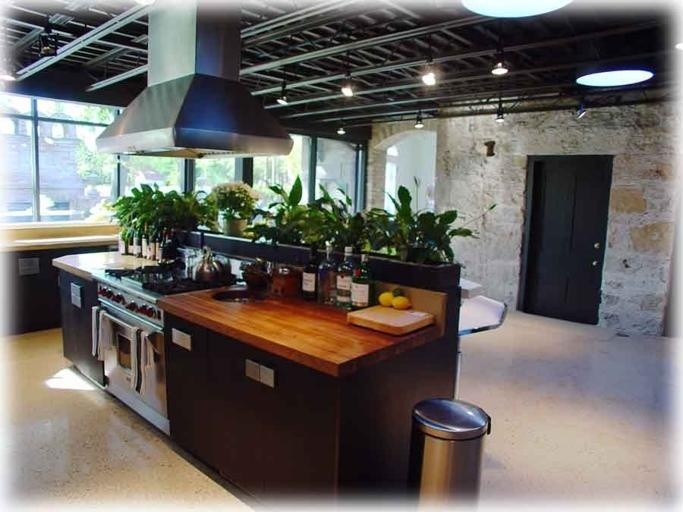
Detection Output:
[221,219,248,237]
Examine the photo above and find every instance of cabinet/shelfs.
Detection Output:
[49,271,104,387]
[163,312,207,465]
[208,328,459,512]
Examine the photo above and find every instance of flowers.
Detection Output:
[213,180,262,219]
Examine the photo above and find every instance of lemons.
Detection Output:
[393,296,410,310]
[378,291,394,306]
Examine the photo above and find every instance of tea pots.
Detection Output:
[188,245,223,283]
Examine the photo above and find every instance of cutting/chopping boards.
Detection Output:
[345,306,435,336]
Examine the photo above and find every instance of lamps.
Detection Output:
[573,101,587,122]
[421,32,438,86]
[36,16,59,58]
[275,64,289,108]
[495,99,505,123]
[339,52,354,97]
[490,19,508,76]
[413,108,424,130]
[337,114,346,136]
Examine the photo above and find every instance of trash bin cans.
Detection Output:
[406,398,491,506]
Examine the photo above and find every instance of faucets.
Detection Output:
[244,263,273,292]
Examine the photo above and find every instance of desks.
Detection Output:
[1,236,120,336]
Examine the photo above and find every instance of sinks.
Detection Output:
[211,289,272,303]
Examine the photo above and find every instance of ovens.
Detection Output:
[89,298,172,436]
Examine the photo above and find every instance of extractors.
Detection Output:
[95,1,295,158]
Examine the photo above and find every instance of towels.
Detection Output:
[88,304,111,362]
[127,324,155,398]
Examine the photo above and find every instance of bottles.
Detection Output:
[333,246,356,311]
[347,249,375,310]
[118,218,177,263]
[314,240,337,307]
[300,241,318,299]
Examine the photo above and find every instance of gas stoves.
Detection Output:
[102,259,209,302]
[119,267,236,296]
